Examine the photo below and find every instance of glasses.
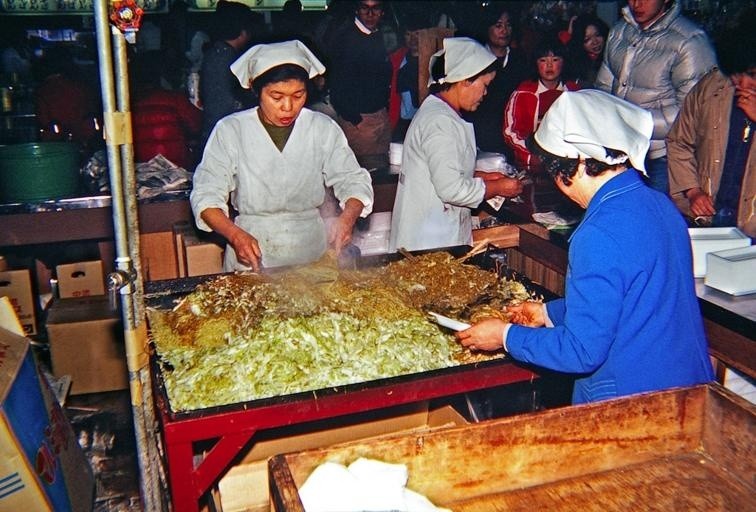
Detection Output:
[356,6,383,16]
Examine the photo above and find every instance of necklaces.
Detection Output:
[741,117,752,143]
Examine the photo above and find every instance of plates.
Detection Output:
[704,246,755,295]
[688,225,750,277]
[431,307,472,339]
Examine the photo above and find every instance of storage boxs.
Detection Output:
[139,232,178,282]
[0,297,95,512]
[44,297,130,396]
[90,241,116,274]
[0,269,37,335]
[186,241,224,277]
[56,260,105,299]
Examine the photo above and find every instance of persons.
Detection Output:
[592,0,718,197]
[501,42,583,170]
[455,89,713,405]
[189,39,375,275]
[0,0,461,172]
[461,6,535,153]
[665,28,756,242]
[389,35,524,251]
[553,13,611,82]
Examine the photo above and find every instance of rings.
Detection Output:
[745,93,751,99]
